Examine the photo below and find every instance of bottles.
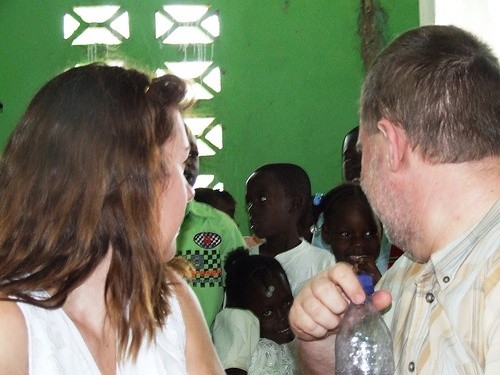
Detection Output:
[336,276,395,375]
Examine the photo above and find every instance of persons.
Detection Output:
[176,124,391,375]
[0,62,229,375]
[287,26,500,375]
